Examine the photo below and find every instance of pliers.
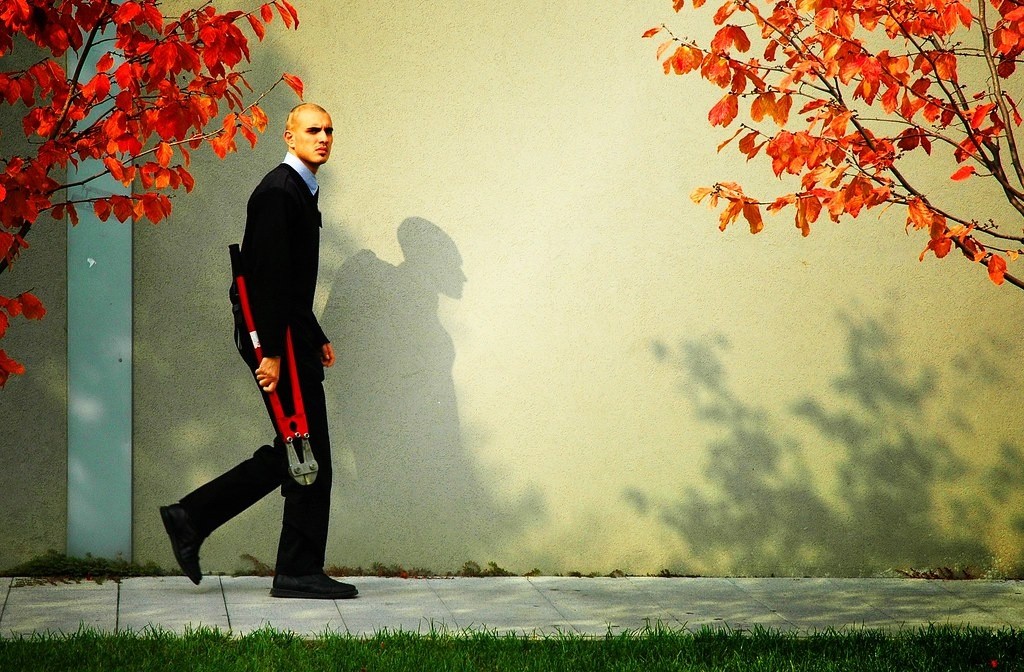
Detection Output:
[228,243,319,486]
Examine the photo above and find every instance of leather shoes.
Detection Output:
[270,567,358,599]
[160,503,205,585]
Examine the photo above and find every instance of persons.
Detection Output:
[157,102,359,599]
